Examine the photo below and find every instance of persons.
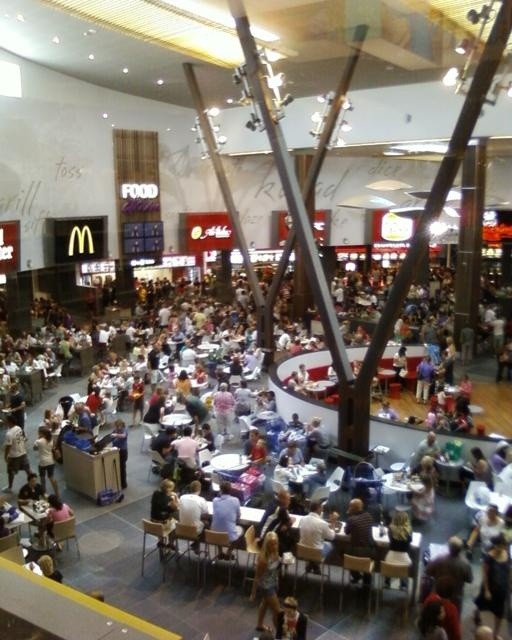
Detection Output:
[1,272,512,640]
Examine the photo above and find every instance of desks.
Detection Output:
[1,501,32,543]
[0,334,464,503]
[20,505,47,545]
[169,502,422,607]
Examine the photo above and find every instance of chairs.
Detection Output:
[376,561,412,630]
[242,525,258,586]
[176,523,207,584]
[203,529,238,587]
[293,543,330,615]
[339,554,374,614]
[141,519,178,582]
[46,516,80,559]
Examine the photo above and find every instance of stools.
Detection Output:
[477,424,486,435]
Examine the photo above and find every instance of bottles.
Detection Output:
[379,521,383,538]
[239,452,243,465]
